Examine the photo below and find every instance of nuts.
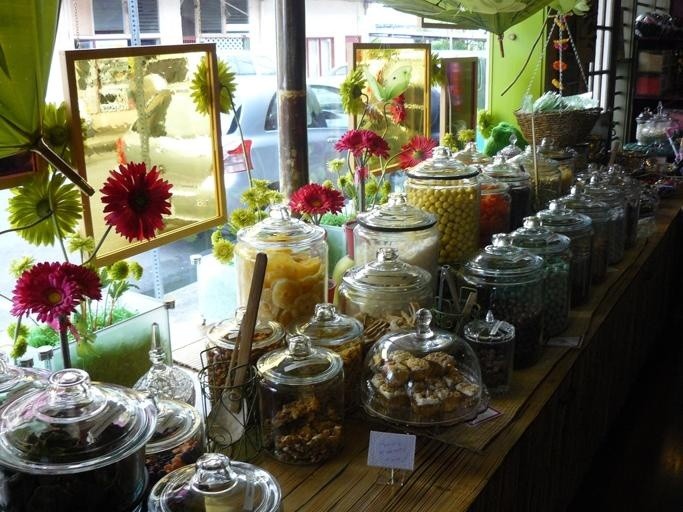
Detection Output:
[239,238,326,334]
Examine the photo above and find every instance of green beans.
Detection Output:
[407,179,476,263]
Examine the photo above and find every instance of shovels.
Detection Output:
[203,252,268,445]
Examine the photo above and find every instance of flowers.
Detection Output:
[0,101,173,358]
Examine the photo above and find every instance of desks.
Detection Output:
[202,192,681,512]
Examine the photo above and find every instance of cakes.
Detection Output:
[367,349,481,416]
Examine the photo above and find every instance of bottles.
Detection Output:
[0,321,282,509]
[236,134,682,391]
[203,302,485,463]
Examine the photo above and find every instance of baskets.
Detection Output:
[513,107,603,146]
[199,347,262,461]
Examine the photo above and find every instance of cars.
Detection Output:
[324,61,439,147]
[115,82,417,241]
